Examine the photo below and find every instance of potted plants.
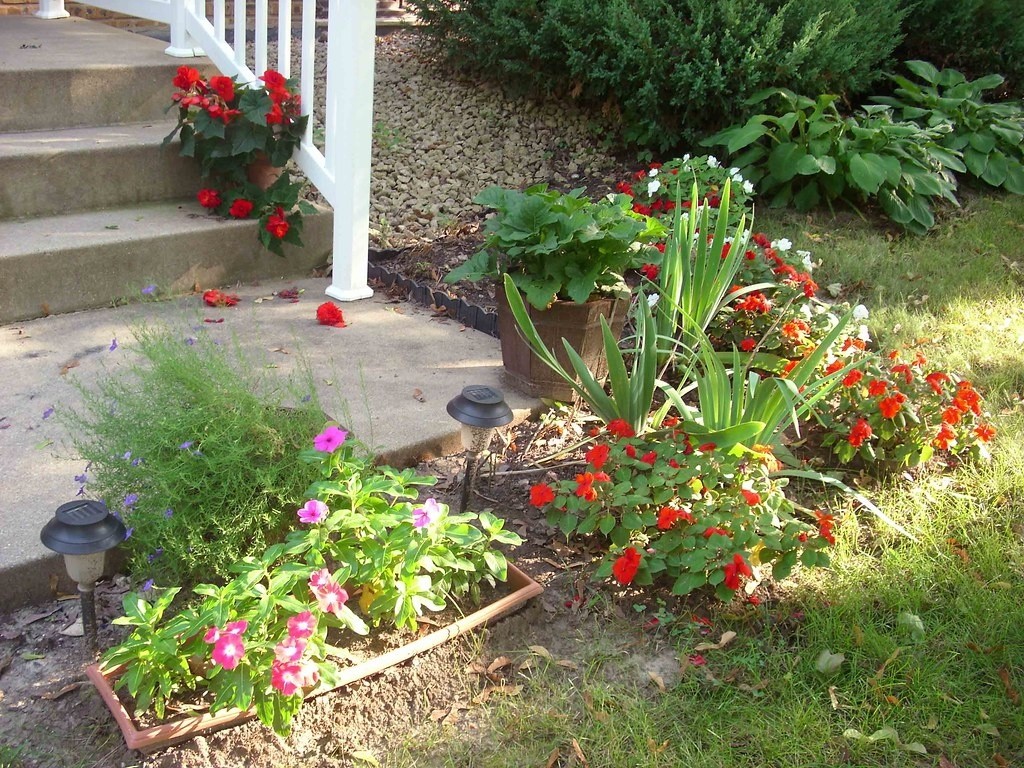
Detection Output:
[441,182,674,402]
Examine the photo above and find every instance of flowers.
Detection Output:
[160,65,319,261]
[22,264,528,737]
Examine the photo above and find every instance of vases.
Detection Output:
[247,156,282,191]
[83,559,545,755]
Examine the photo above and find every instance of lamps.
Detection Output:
[446,384,514,513]
[42,501,126,648]
